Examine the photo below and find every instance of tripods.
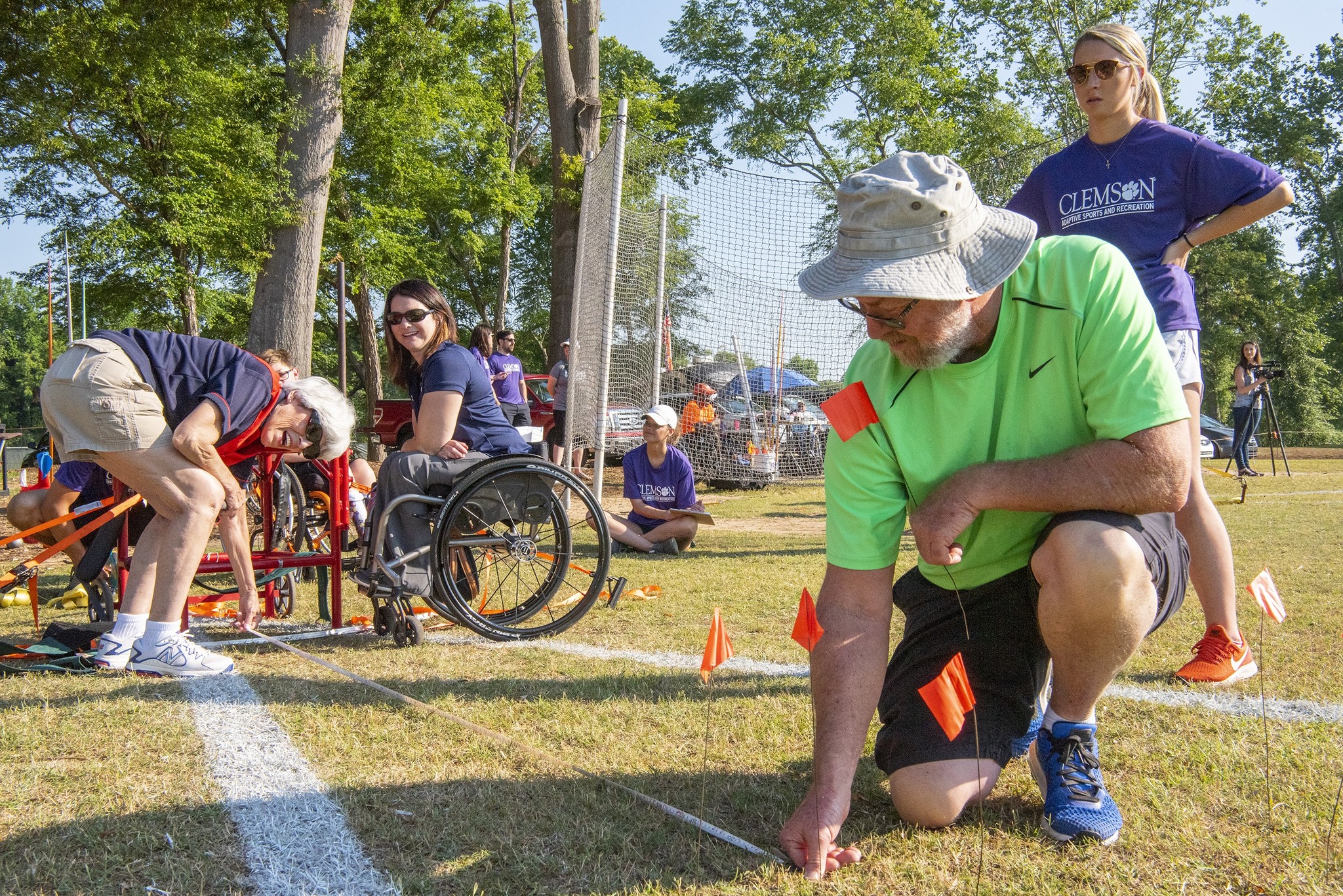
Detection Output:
[1225,379,1292,478]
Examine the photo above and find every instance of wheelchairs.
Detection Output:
[357,410,612,650]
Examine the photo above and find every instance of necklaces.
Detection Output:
[1089,116,1139,169]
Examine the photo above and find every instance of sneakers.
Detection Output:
[108,553,118,602]
[124,636,235,677]
[1174,623,1258,686]
[1027,723,1124,847]
[348,570,431,597]
[96,629,145,670]
[1238,468,1265,477]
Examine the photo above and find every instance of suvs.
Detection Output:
[1200,413,1259,458]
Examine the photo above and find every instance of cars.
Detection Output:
[20,430,62,469]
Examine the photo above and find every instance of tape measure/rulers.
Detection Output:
[246,624,802,871]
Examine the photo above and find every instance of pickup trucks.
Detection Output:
[369,372,647,471]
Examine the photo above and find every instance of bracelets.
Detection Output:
[1183,233,1195,248]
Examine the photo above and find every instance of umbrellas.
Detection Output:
[714,365,820,399]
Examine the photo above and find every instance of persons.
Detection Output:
[348,279,532,596]
[586,404,706,558]
[547,338,592,481]
[682,383,723,471]
[7,461,157,587]
[467,324,512,407]
[1233,340,1268,477]
[779,151,1191,880]
[43,327,357,678]
[256,349,377,556]
[790,400,820,440]
[1007,23,1295,686]
[487,331,532,427]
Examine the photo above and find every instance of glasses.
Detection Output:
[389,308,434,325]
[501,338,516,342]
[798,406,804,408]
[563,345,569,348]
[1243,339,1258,348]
[488,333,494,338]
[301,408,323,458]
[1069,60,1132,85]
[279,369,293,381]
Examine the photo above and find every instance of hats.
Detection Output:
[692,383,717,396]
[560,339,570,347]
[797,151,1038,301]
[797,399,805,405]
[640,404,678,430]
